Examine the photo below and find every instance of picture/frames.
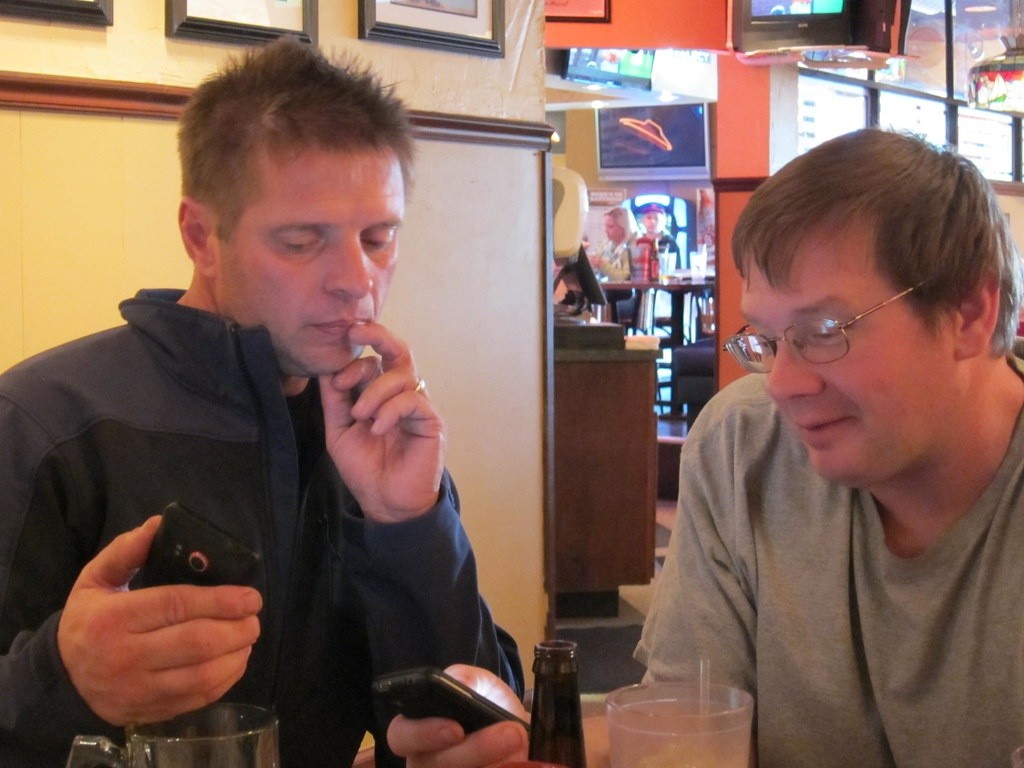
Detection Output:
[545,0,611,23]
[0,0,115,26]
[358,0,505,58]
[165,0,319,49]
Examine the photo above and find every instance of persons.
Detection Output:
[631,129,1022,768]
[0,45,526,768]
[628,202,684,285]
[591,206,633,283]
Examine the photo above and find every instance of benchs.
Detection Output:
[674,337,716,434]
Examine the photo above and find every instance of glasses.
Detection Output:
[722,281,924,374]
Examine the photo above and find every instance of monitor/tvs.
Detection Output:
[595,103,711,183]
[559,245,607,315]
[732,0,854,53]
[561,47,655,91]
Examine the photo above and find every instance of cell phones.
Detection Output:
[141,502,264,586]
[371,666,533,736]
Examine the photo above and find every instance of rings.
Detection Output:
[413,376,426,394]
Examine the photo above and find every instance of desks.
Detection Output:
[599,280,714,421]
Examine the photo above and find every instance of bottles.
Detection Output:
[529,639,589,768]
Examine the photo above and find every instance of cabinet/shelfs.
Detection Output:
[555,302,664,618]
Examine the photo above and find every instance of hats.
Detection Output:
[635,194,670,213]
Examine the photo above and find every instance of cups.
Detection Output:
[658,252,676,285]
[67,701,279,768]
[690,250,707,284]
[604,681,754,768]
